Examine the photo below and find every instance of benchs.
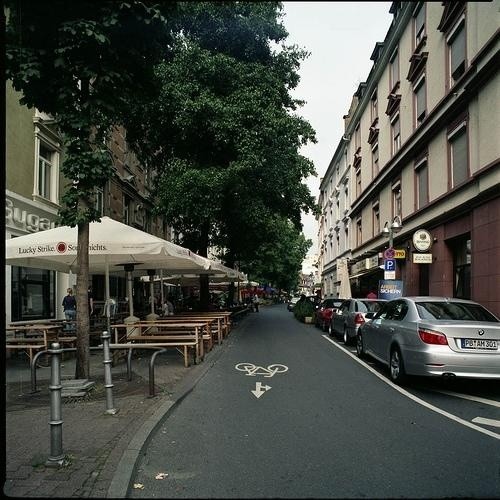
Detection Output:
[5,309,231,368]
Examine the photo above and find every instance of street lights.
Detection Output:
[381,215,402,249]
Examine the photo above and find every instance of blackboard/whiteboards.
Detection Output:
[378,279,403,298]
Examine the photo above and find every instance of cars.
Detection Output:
[287,297,301,311]
[315,296,500,382]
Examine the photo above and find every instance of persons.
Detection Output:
[367,289,377,299]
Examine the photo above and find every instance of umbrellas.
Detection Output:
[5,215,259,344]
